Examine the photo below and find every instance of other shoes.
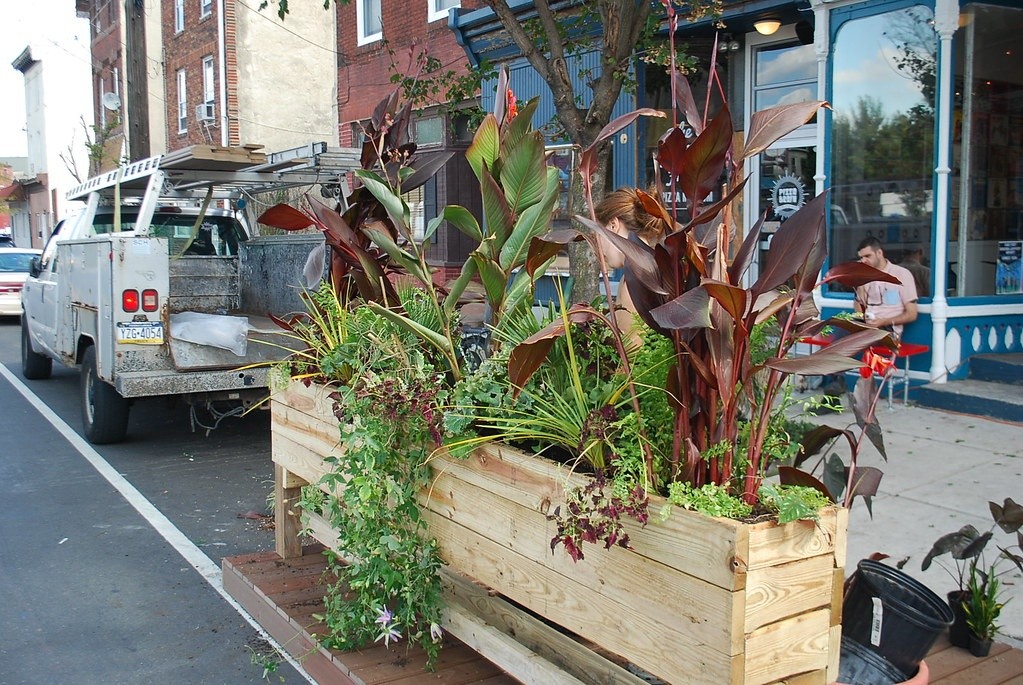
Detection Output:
[807,398,842,415]
[824,378,847,395]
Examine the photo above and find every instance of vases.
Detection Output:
[268,376,842,683]
[837,558,947,684]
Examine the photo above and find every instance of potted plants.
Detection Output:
[922,498,1022,652]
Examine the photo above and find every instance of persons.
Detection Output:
[588,185,705,352]
[899,237,931,297]
[808,237,920,416]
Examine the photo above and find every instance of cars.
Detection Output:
[0,234,25,269]
[0,247,45,315]
[828,178,933,273]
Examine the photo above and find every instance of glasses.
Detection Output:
[866,286,882,306]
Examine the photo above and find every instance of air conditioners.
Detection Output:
[195,101,214,121]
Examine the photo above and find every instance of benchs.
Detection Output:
[790,330,931,408]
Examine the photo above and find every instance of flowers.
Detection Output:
[250,0,897,684]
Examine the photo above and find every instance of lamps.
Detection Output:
[756,14,785,36]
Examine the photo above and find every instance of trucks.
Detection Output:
[19,166,355,446]
